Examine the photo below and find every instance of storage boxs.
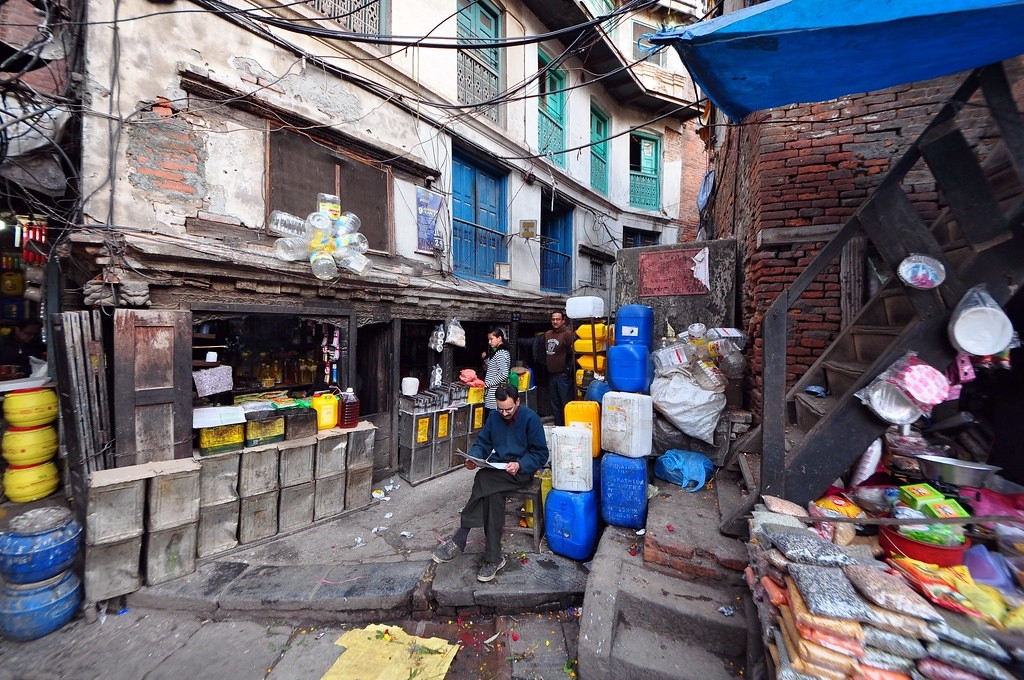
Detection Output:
[285,410,318,440]
[898,484,972,517]
[244,405,286,447]
[192,405,247,456]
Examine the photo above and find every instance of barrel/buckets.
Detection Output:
[878,513,972,568]
[1,507,83,642]
[1,388,60,503]
[527,296,658,558]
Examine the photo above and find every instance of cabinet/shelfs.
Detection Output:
[0,251,46,380]
[192,320,317,403]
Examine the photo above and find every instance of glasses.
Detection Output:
[496,403,516,413]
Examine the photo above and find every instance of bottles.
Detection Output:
[650,322,747,391]
[268,193,372,279]
[338,388,359,428]
[509,371,518,388]
[236,351,317,388]
[312,393,338,430]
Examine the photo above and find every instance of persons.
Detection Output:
[0,318,48,368]
[482,330,511,424]
[432,384,549,582]
[518,311,575,426]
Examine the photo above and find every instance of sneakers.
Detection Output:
[432,540,463,563]
[477,557,506,581]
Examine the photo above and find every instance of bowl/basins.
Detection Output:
[900,256,946,289]
[869,382,921,425]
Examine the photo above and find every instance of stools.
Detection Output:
[505,476,545,552]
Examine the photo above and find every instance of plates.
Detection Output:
[905,365,949,405]
[953,307,1013,356]
